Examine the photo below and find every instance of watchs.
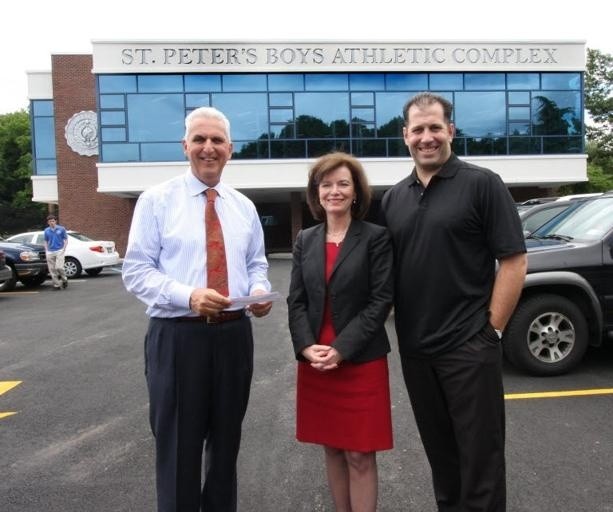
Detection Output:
[493,329,503,340]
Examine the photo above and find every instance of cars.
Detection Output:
[0,230,120,279]
[0,249,14,281]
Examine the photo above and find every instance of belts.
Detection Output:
[182,311,242,324]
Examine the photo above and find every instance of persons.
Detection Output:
[376,95,528,512]
[286,152,396,512]
[43,215,69,289]
[121,105,275,512]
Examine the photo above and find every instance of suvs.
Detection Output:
[0,235,48,292]
[511,192,605,240]
[493,191,612,377]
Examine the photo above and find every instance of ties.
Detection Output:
[205,189,229,297]
